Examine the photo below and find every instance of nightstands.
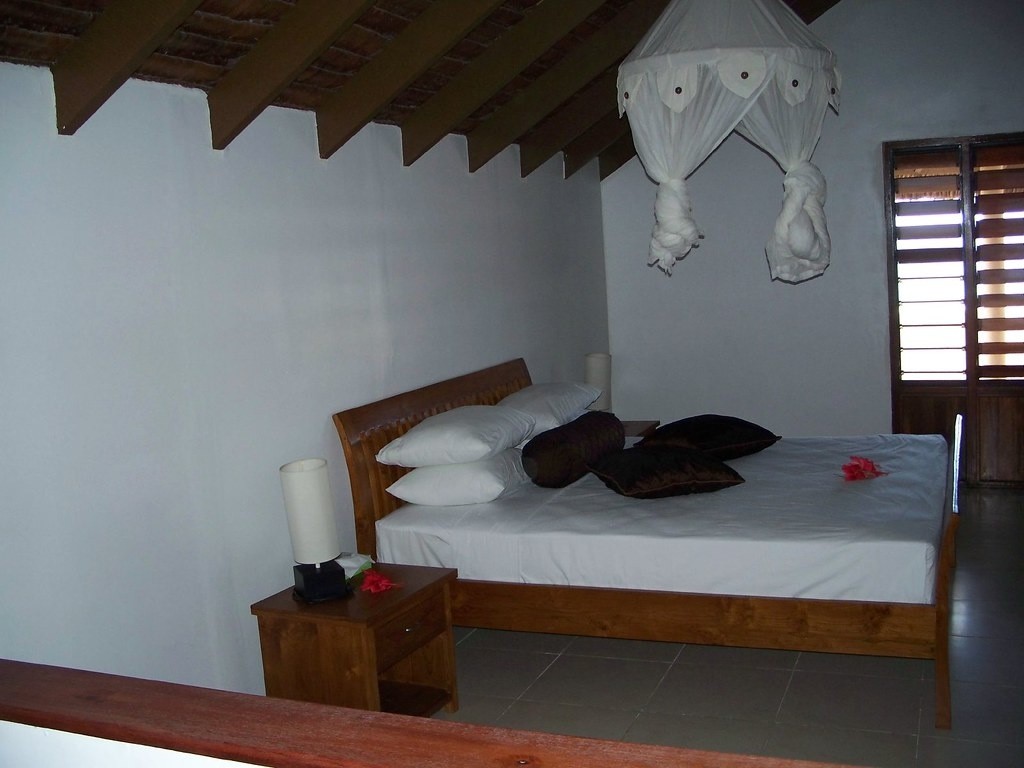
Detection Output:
[620,419,662,437]
[249,560,461,723]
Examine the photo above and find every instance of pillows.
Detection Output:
[632,413,783,463]
[583,447,747,501]
[382,442,531,506]
[373,402,538,470]
[495,377,601,445]
[521,409,626,490]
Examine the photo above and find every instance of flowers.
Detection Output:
[360,569,404,593]
[838,452,889,482]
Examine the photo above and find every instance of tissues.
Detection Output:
[335,552,373,580]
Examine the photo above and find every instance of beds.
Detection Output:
[327,355,965,736]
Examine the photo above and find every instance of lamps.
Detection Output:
[276,452,352,608]
[583,351,613,412]
